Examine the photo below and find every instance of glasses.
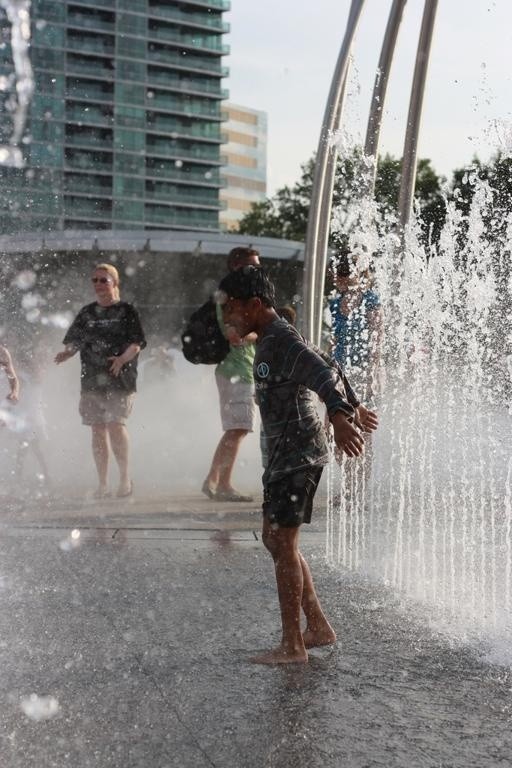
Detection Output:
[91,276,112,285]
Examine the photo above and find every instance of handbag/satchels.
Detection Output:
[181,299,230,364]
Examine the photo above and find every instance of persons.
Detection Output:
[322,243,388,517]
[214,265,381,669]
[52,263,147,500]
[275,306,298,327]
[10,345,59,504]
[0,342,19,403]
[202,244,269,502]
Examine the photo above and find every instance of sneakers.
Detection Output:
[206,484,253,502]
